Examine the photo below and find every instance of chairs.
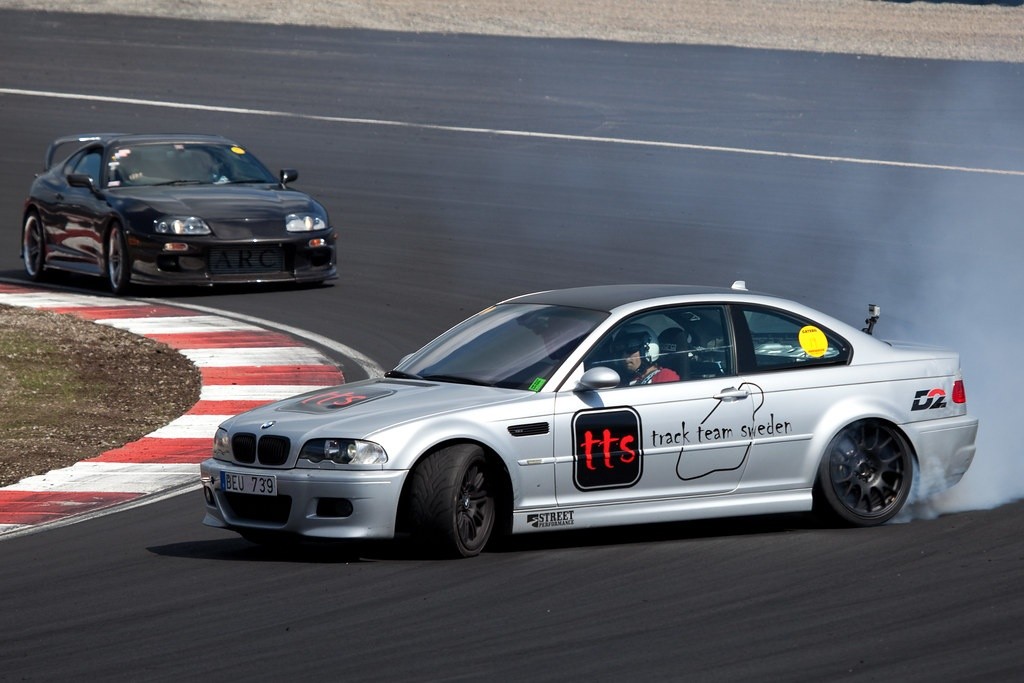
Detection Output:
[656,327,691,383]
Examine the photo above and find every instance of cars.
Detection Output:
[202,279,982,560]
[18,133,339,296]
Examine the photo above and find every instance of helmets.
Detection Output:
[614,322,661,363]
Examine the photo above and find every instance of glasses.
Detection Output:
[613,345,646,359]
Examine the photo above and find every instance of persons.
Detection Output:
[609,322,681,388]
